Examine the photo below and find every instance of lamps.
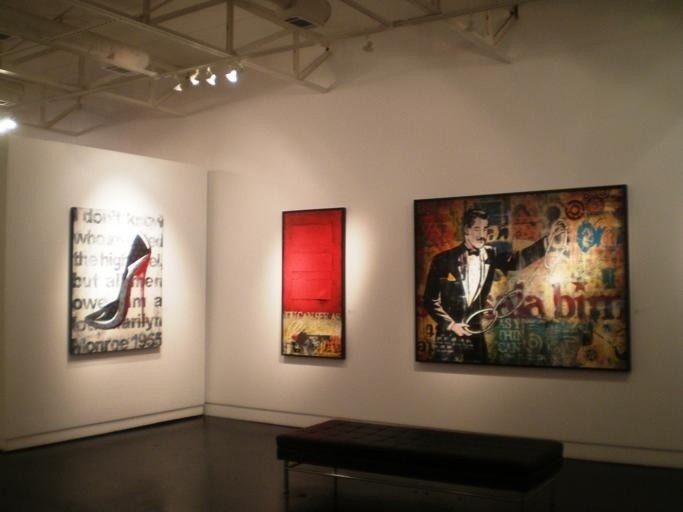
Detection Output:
[172,54,243,92]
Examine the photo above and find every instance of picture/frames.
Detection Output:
[281,208,345,360]
[414,183,631,372]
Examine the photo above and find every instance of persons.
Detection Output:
[424,208,569,365]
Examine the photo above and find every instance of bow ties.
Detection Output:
[466,249,480,256]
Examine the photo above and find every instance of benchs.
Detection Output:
[276,420,563,512]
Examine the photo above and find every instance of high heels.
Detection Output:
[84,235,149,328]
